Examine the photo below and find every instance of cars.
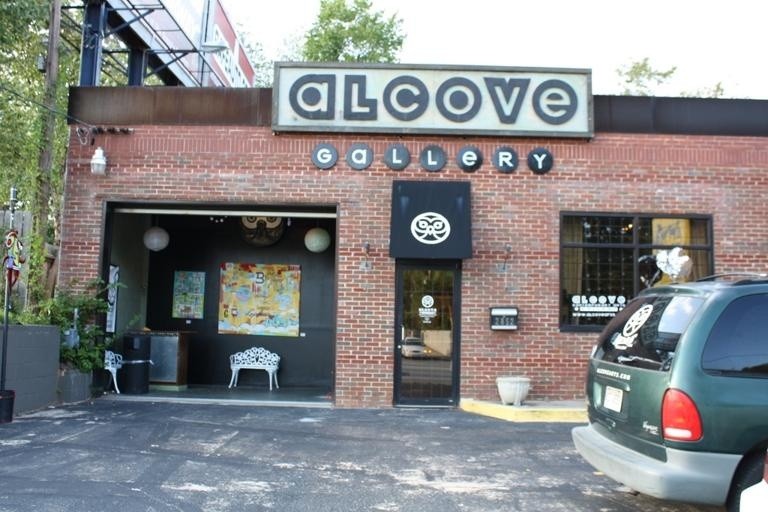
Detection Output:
[401,337,425,360]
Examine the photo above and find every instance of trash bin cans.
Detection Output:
[123,331,150,395]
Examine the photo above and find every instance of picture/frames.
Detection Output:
[106,263,119,334]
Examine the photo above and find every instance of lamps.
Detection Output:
[88,147,107,177]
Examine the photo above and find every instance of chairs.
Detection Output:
[228,347,280,391]
[104,350,124,394]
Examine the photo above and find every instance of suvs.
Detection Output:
[569,268,768,512]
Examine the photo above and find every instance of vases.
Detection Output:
[497,376,530,404]
